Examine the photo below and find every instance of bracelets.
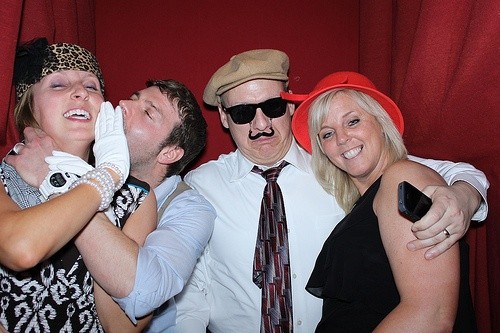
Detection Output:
[68,162,124,212]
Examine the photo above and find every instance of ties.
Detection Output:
[251,160,294,333]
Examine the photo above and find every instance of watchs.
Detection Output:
[38,171,79,200]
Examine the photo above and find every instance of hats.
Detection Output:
[12,38,105,101]
[280,71,404,155]
[202,49,289,106]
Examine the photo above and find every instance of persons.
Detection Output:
[4,79,217,333]
[0,39,158,333]
[175,50,491,333]
[291,73,475,333]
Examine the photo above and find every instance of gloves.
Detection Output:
[92,101,130,183]
[45,150,117,227]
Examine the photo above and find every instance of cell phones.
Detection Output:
[397,181,433,223]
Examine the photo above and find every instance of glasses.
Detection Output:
[219,97,288,124]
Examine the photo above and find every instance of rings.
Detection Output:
[444,229,450,238]
